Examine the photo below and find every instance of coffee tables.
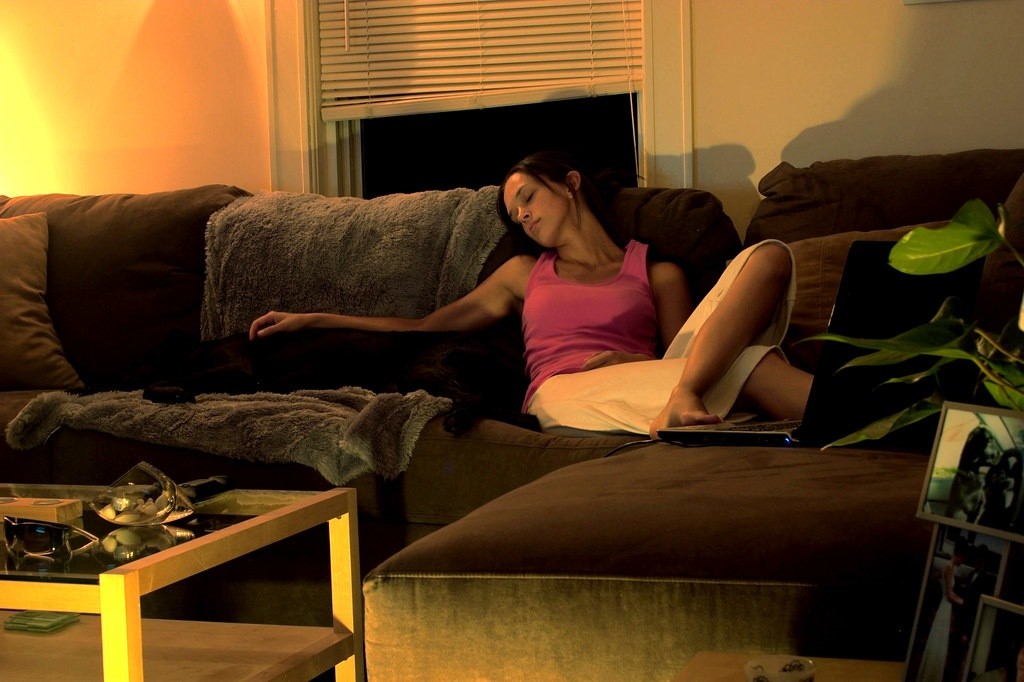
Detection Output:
[0,483,366,682]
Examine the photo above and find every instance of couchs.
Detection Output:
[0,148,1024,682]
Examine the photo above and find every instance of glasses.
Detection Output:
[2,515,100,557]
[5,549,93,575]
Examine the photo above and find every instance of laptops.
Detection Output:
[655,241,985,451]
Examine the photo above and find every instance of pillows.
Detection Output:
[0,209,87,392]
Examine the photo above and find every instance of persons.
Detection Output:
[916,448,1024,682]
[249,150,815,440]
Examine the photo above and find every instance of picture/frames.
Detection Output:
[900,524,1012,682]
[913,401,1024,543]
